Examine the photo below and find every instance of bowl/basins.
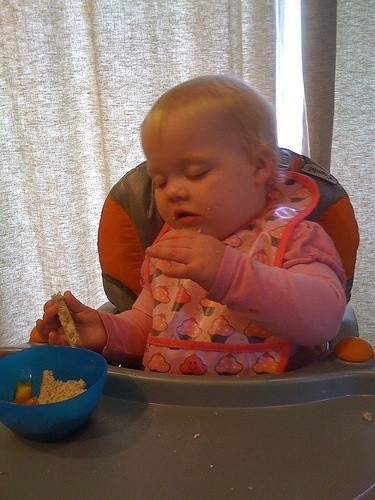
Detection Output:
[0,346,107,442]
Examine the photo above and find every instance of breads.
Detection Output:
[50,292,82,347]
[37,370,87,404]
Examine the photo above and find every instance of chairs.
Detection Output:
[94,147,361,338]
